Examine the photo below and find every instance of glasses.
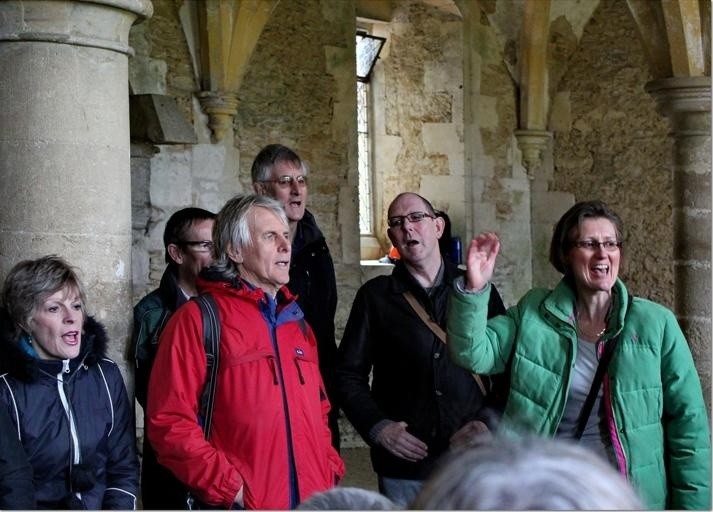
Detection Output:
[258,175,308,188]
[574,240,622,249]
[176,241,213,249]
[388,212,435,226]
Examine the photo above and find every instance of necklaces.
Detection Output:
[573,302,609,341]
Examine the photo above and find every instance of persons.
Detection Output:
[250,143,340,490]
[130,208,218,510]
[442,203,711,509]
[146,193,344,510]
[0,251,140,509]
[289,483,403,510]
[330,189,512,509]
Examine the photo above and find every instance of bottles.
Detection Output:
[451,236,462,263]
